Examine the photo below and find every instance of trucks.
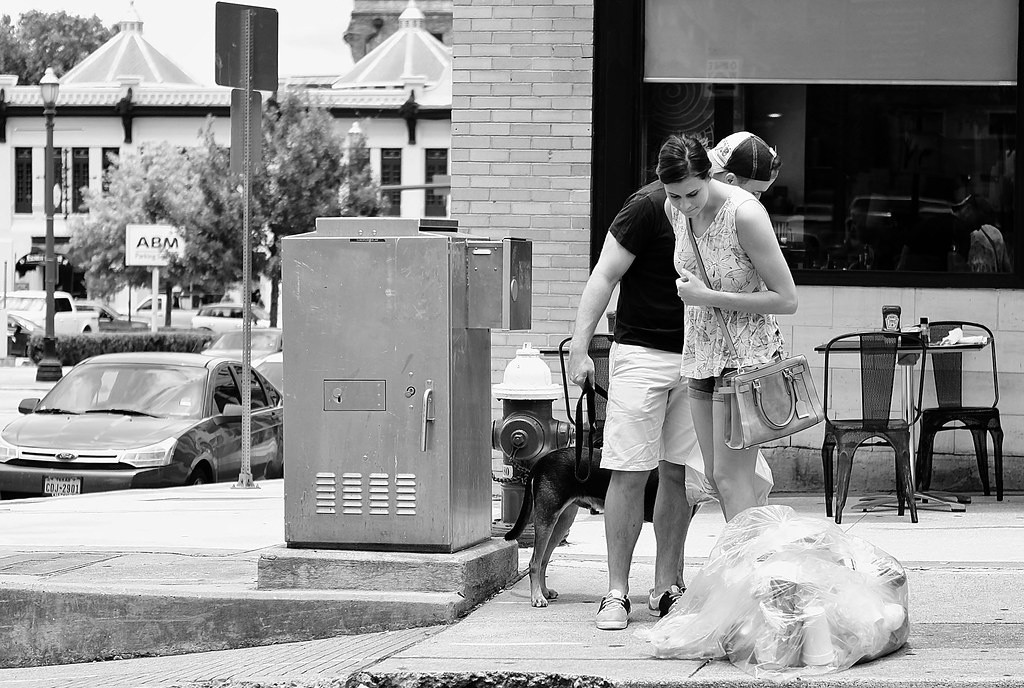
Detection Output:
[135,293,199,325]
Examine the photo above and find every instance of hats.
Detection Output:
[707,131,780,181]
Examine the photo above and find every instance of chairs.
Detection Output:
[820,332,918,523]
[558,332,616,448]
[915,321,1005,501]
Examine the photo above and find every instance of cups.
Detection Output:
[802,606,835,665]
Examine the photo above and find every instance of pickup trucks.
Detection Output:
[1,290,99,336]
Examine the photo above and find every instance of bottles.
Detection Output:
[881,305,902,344]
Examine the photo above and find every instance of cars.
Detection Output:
[191,302,270,333]
[7,314,46,357]
[74,301,152,332]
[199,328,285,393]
[0,351,283,495]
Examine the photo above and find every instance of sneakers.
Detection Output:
[647,584,683,617]
[596,588,632,629]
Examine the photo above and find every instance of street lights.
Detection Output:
[36,67,63,381]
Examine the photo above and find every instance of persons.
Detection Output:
[567,132,784,630]
[656,135,798,525]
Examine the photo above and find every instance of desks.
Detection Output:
[813,337,990,513]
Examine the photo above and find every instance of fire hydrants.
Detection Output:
[492,341,574,548]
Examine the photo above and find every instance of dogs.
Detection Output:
[503,446,702,608]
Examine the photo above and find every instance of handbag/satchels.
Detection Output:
[716,352,824,450]
